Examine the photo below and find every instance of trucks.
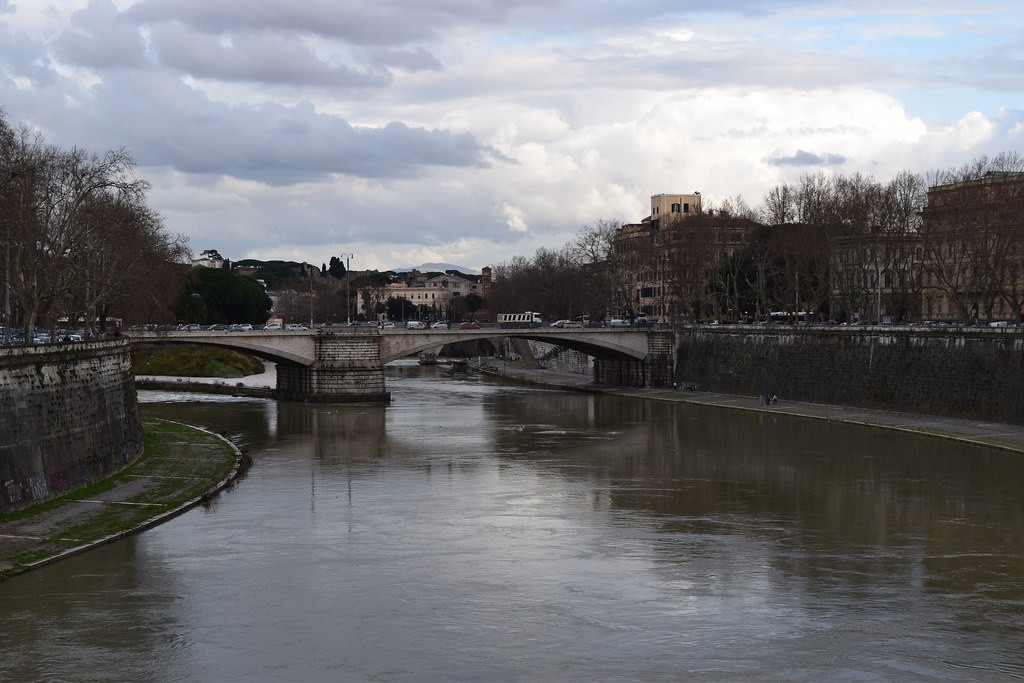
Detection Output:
[496,310,542,323]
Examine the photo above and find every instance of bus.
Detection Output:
[766,311,814,326]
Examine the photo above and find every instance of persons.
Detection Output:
[99,330,107,343]
[187,325,191,333]
[760,393,778,406]
[674,381,677,391]
[381,320,384,330]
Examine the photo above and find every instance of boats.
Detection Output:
[445,359,468,381]
[418,352,442,366]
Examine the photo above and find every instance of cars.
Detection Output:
[549,319,582,329]
[128,322,254,332]
[286,323,309,332]
[458,322,480,329]
[378,322,396,330]
[0,325,85,349]
[262,322,282,330]
[433,321,451,330]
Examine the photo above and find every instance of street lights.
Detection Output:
[340,252,354,326]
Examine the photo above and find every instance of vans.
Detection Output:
[407,321,426,330]
[610,315,638,328]
